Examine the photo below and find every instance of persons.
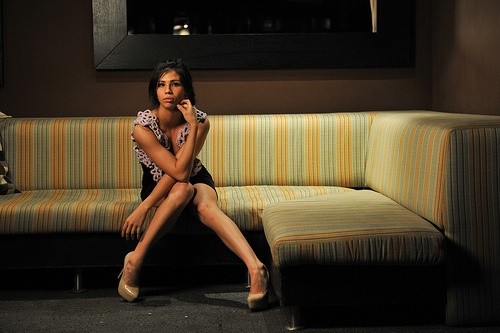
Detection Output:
[115,59,270,310]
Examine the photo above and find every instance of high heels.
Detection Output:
[118,251,138,301]
[247,266,272,309]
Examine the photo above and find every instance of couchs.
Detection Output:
[0,110,500,333]
